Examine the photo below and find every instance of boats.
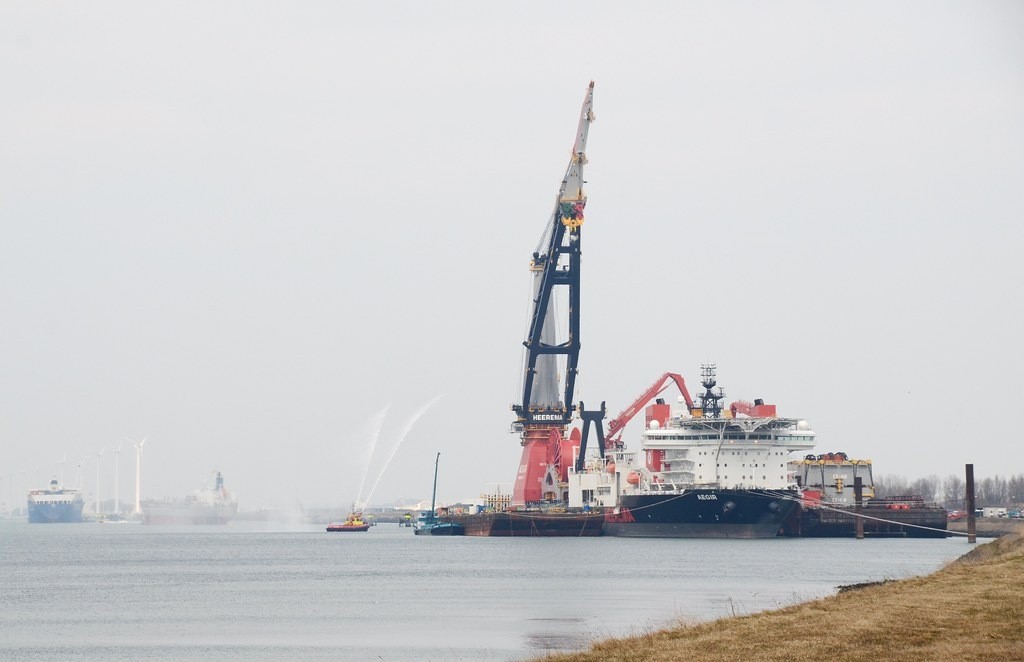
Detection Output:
[26,476,88,523]
[566,360,816,539]
[139,473,239,524]
[788,451,948,539]
[398,81,604,537]
[326,503,377,532]
